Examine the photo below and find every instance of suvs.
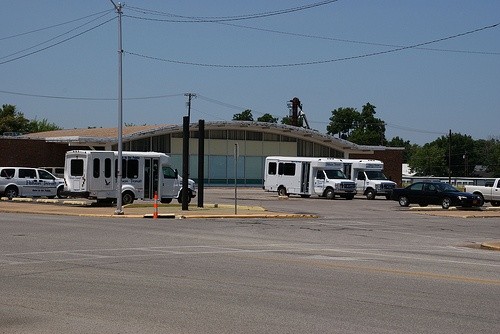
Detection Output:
[0,167,69,199]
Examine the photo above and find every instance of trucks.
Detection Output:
[262,156,358,200]
[350,159,398,200]
[63,149,197,204]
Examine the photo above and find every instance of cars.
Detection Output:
[390,181,482,210]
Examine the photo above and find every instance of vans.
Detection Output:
[39,166,64,178]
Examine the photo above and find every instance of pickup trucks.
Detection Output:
[464,177,500,206]
